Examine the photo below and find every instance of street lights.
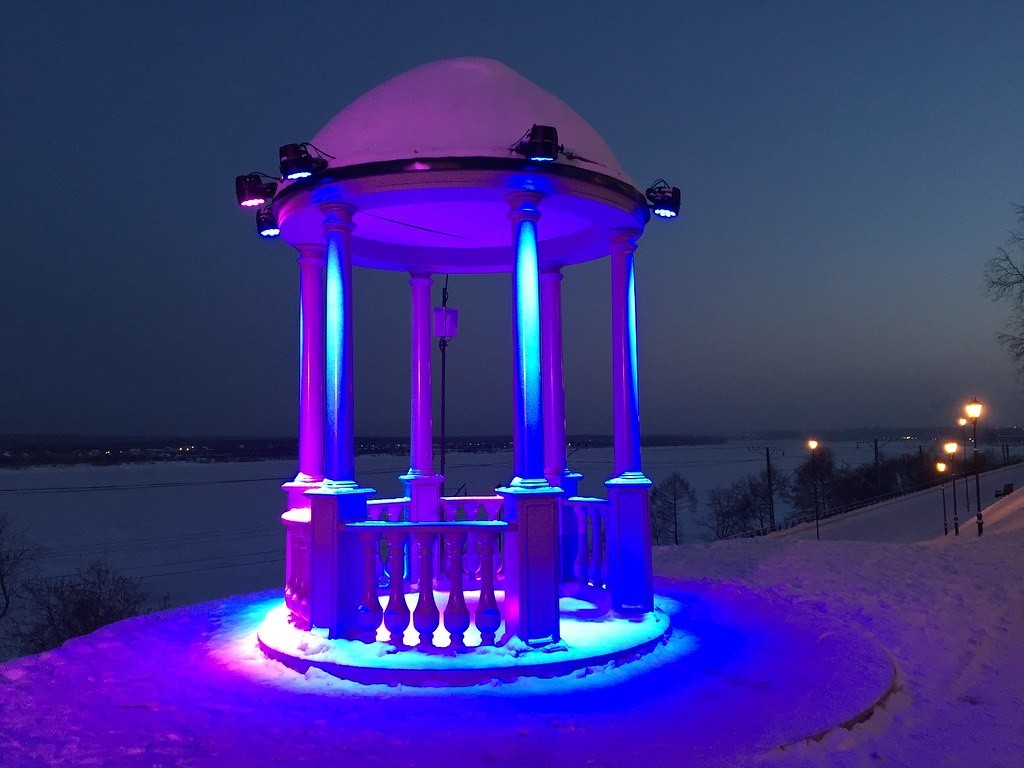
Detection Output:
[943,441,959,538]
[960,416,969,512]
[432,275,461,574]
[966,397,984,535]
[935,460,949,534]
[808,439,820,539]
